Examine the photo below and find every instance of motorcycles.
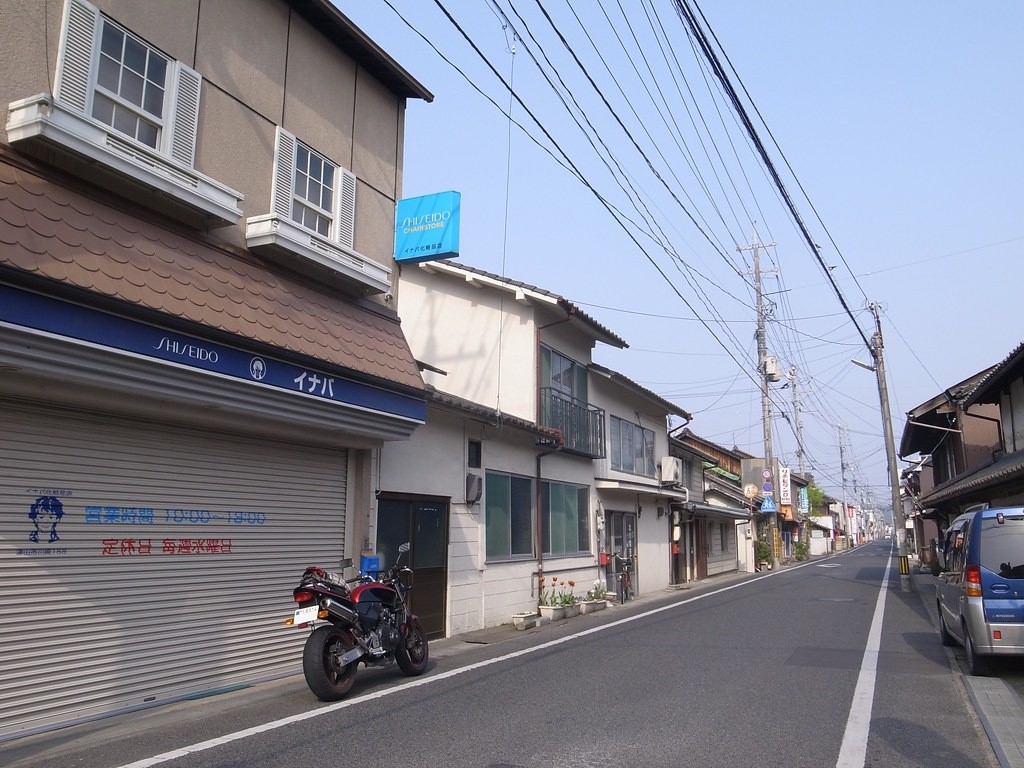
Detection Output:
[285,542,429,700]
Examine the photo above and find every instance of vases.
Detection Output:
[539,606,565,620]
[557,603,581,618]
[594,600,607,610]
[579,600,596,613]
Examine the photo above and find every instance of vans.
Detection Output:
[929,502,1024,676]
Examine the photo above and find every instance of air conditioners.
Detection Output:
[662,457,683,484]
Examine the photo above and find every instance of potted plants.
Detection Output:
[512,611,538,626]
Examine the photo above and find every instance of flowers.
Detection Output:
[539,577,607,605]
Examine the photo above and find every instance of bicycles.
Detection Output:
[611,552,637,604]
